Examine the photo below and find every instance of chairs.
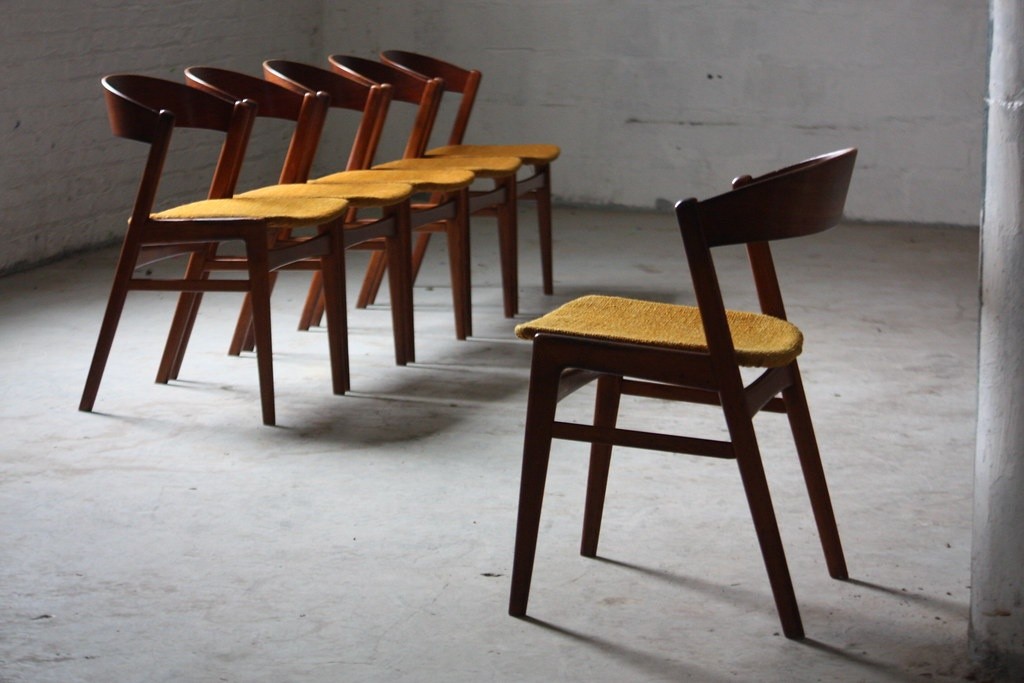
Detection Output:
[380,49,560,313]
[507,148,859,642]
[183,66,409,391]
[262,58,473,362]
[327,53,523,336]
[81,74,346,425]
